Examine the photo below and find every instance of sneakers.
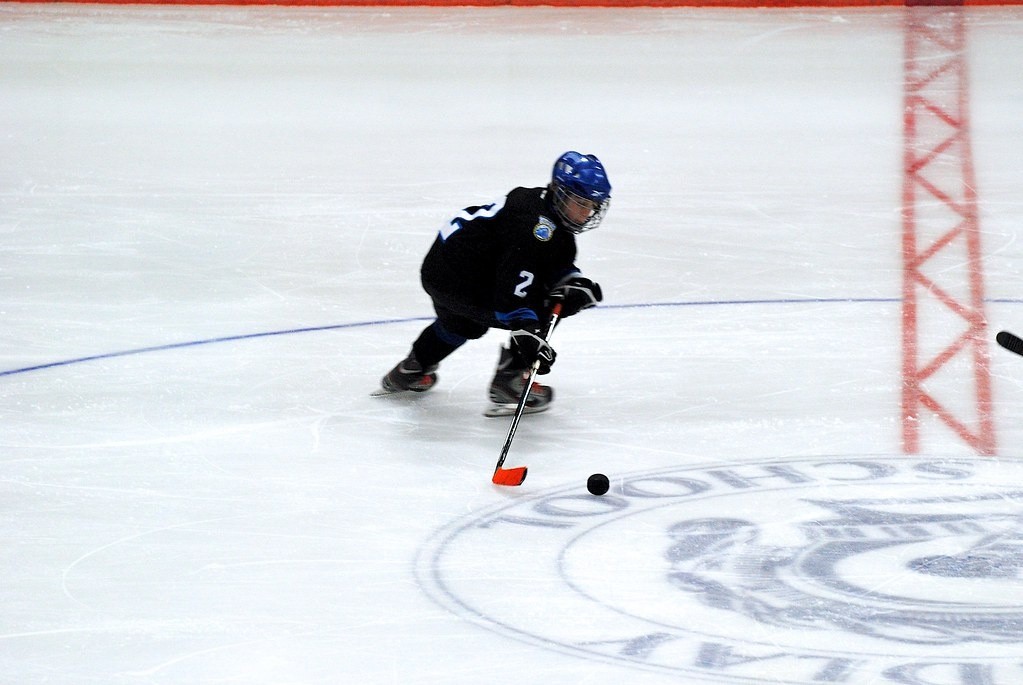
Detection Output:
[370,350,439,397]
[483,347,554,417]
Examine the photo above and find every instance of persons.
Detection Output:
[368,151,612,422]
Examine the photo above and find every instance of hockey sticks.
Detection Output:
[996,331,1023,356]
[491,301,562,487]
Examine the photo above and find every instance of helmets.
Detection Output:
[551,152,611,236]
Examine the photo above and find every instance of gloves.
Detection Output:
[549,275,603,319]
[510,319,557,375]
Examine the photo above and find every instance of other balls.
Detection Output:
[587,473,610,496]
